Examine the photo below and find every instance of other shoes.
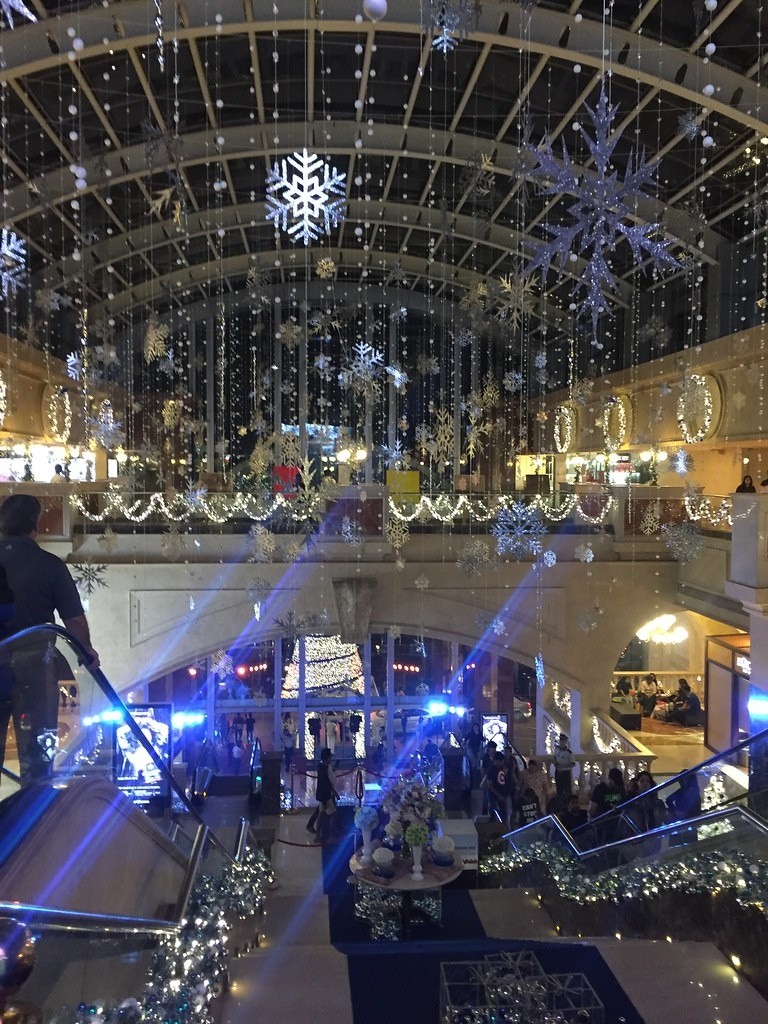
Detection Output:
[306,825,316,834]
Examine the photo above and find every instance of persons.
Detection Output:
[321,470,336,486]
[306,748,341,844]
[552,734,576,795]
[736,475,756,493]
[51,464,66,483]
[516,760,549,815]
[480,746,520,817]
[400,710,485,764]
[548,795,591,851]
[662,678,700,722]
[616,675,633,706]
[309,711,350,754]
[666,769,701,844]
[0,494,100,789]
[587,767,667,869]
[116,718,169,780]
[480,741,497,816]
[22,464,32,481]
[510,789,541,841]
[219,712,293,776]
[640,673,657,715]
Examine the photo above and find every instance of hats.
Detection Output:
[645,675,653,681]
[559,733,569,741]
[320,747,334,760]
[0,495,42,538]
[493,753,504,760]
[487,741,497,750]
[608,769,625,788]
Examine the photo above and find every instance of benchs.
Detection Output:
[610,701,642,731]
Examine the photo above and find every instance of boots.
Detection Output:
[314,811,342,844]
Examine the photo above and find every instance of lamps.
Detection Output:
[636,614,688,646]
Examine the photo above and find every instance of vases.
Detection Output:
[361,828,372,863]
[377,860,393,869]
[437,851,451,860]
[389,834,403,850]
[412,845,424,882]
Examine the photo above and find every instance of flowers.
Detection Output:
[433,835,456,853]
[354,807,378,830]
[381,773,445,833]
[385,819,403,837]
[373,848,394,863]
[405,823,430,847]
